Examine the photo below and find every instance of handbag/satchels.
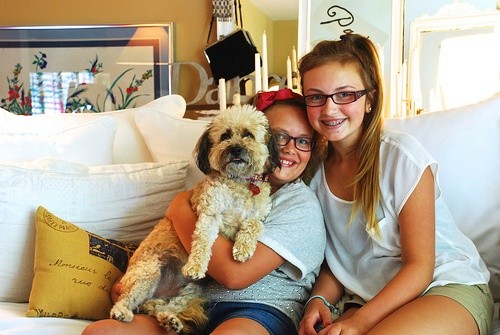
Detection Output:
[203,0,263,86]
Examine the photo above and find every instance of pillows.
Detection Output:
[25,206,139,320]
[381,94,500,279]
[0,160,190,303]
[0,95,187,159]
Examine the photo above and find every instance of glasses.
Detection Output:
[270,131,316,152]
[303,89,377,107]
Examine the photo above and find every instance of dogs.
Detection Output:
[110,104,272,334]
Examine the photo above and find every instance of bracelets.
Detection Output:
[302,295,344,319]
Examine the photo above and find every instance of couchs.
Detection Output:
[0,94,500,335]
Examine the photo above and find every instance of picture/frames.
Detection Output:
[0,23,175,116]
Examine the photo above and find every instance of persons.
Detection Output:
[296,34,495,335]
[81,89,327,335]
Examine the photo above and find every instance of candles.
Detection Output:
[255,54,261,93]
[262,31,268,91]
[287,56,292,89]
[219,78,227,110]
[292,45,297,71]
